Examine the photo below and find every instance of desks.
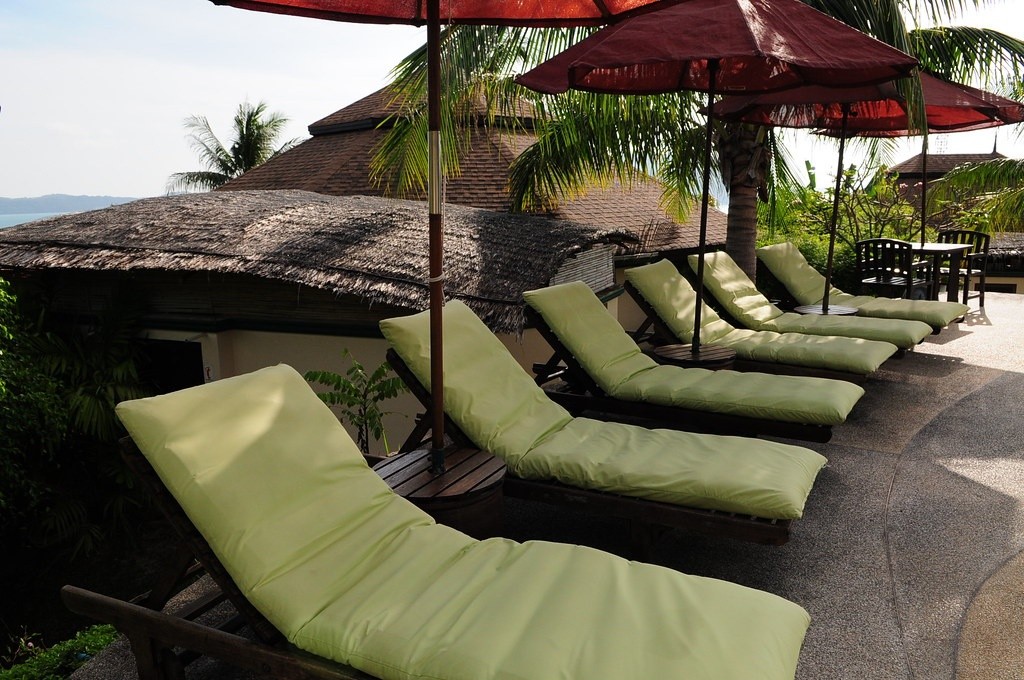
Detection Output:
[372,447,508,539]
[653,343,737,371]
[793,304,860,316]
[871,243,975,303]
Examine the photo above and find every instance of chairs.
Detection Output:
[916,230,991,307]
[60,363,812,680]
[757,242,970,335]
[378,299,829,547]
[855,238,935,300]
[623,258,898,384]
[521,280,866,444]
[688,251,933,360]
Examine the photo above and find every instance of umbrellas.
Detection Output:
[697,69,1024,310]
[515,0,919,345]
[204,0,655,477]
